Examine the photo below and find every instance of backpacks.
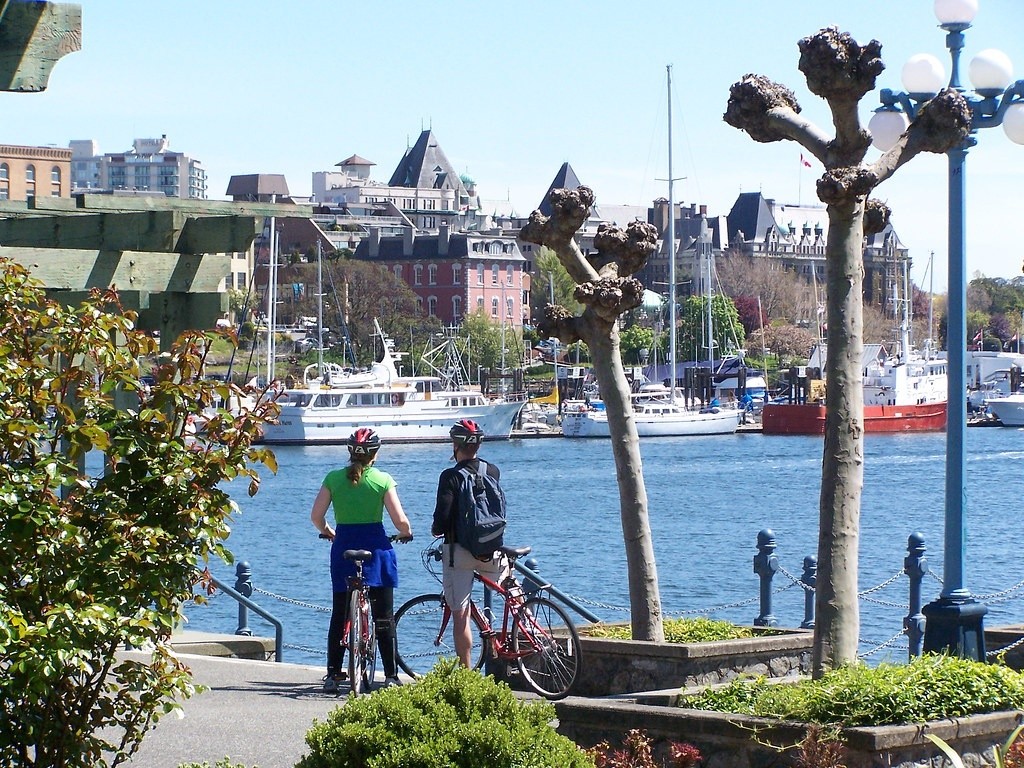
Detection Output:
[454,460,507,557]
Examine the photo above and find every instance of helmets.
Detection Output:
[345,428,383,457]
[446,420,484,443]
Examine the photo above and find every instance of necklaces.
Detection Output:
[311,427,412,694]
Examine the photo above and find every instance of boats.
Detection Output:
[555,357,745,436]
[190,197,537,444]
[758,257,948,432]
[982,380,1024,427]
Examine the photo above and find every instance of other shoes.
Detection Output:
[384,676,404,687]
[323,678,341,691]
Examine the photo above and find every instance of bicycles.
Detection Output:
[318,532,413,699]
[391,536,585,701]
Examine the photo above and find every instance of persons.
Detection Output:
[432,417,535,671]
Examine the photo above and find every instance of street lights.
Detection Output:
[866,0,1024,674]
